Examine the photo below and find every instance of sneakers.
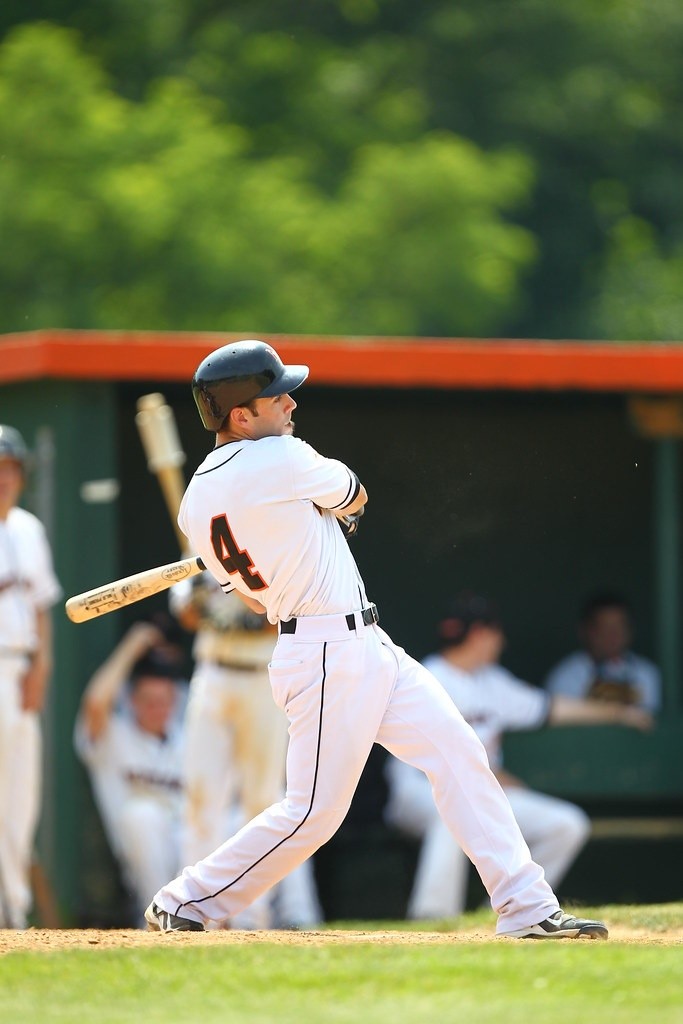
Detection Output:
[144,901,204,933]
[494,912,608,941]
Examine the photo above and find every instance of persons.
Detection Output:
[142,338,609,940]
[72,623,192,939]
[546,577,660,735]
[0,424,64,935]
[385,595,592,929]
[174,528,325,928]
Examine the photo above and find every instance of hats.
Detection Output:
[1,425,31,464]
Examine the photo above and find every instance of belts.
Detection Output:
[363,603,380,626]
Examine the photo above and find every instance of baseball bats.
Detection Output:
[62,502,367,629]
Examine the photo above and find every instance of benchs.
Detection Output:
[501,720,683,839]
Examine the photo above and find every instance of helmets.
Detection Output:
[189,339,310,433]
